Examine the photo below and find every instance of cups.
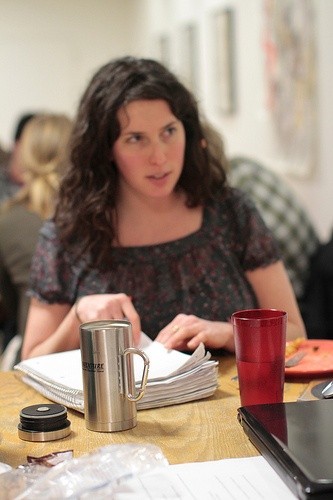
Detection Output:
[231,308,288,407]
[79,319,150,432]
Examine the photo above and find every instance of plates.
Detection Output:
[285,339,333,379]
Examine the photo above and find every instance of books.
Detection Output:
[13,331,220,415]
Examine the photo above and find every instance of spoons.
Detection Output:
[231,353,304,381]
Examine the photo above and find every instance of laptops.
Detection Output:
[235,396,333,500]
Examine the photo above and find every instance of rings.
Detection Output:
[172,325,178,331]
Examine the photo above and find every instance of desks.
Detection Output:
[0,354,310,500]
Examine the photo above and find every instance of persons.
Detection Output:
[198,117,320,299]
[0,112,76,349]
[22,56,307,355]
